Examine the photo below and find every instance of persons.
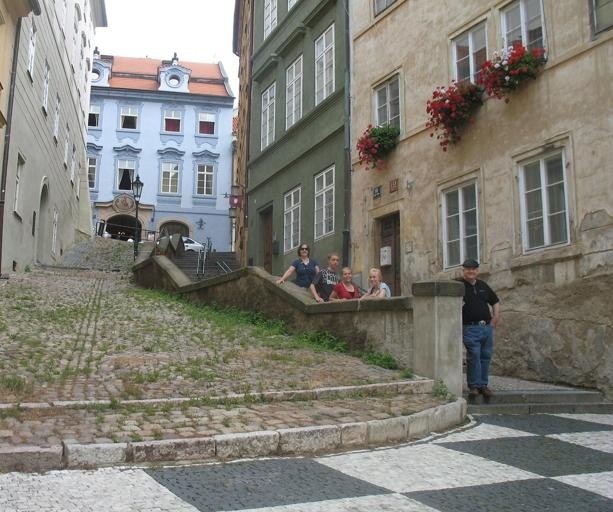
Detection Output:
[461,260,500,400]
[361,268,393,300]
[276,242,320,289]
[328,266,359,301]
[309,253,341,302]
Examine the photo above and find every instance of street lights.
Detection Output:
[131,175,144,262]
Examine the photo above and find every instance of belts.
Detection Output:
[465,320,489,327]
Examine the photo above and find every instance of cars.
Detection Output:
[156,234,205,252]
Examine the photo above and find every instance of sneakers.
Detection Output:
[479,385,491,396]
[469,388,479,396]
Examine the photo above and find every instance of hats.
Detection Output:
[463,259,479,267]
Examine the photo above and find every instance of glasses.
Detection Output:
[301,248,308,250]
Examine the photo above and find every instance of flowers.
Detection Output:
[358,124,399,169]
[423,41,551,150]
